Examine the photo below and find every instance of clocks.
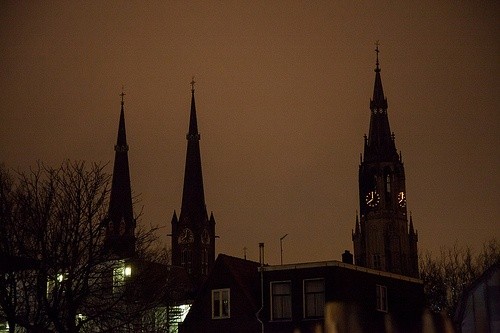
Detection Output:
[366,191,380,207]
[201,229,211,244]
[180,230,192,243]
[398,191,406,207]
